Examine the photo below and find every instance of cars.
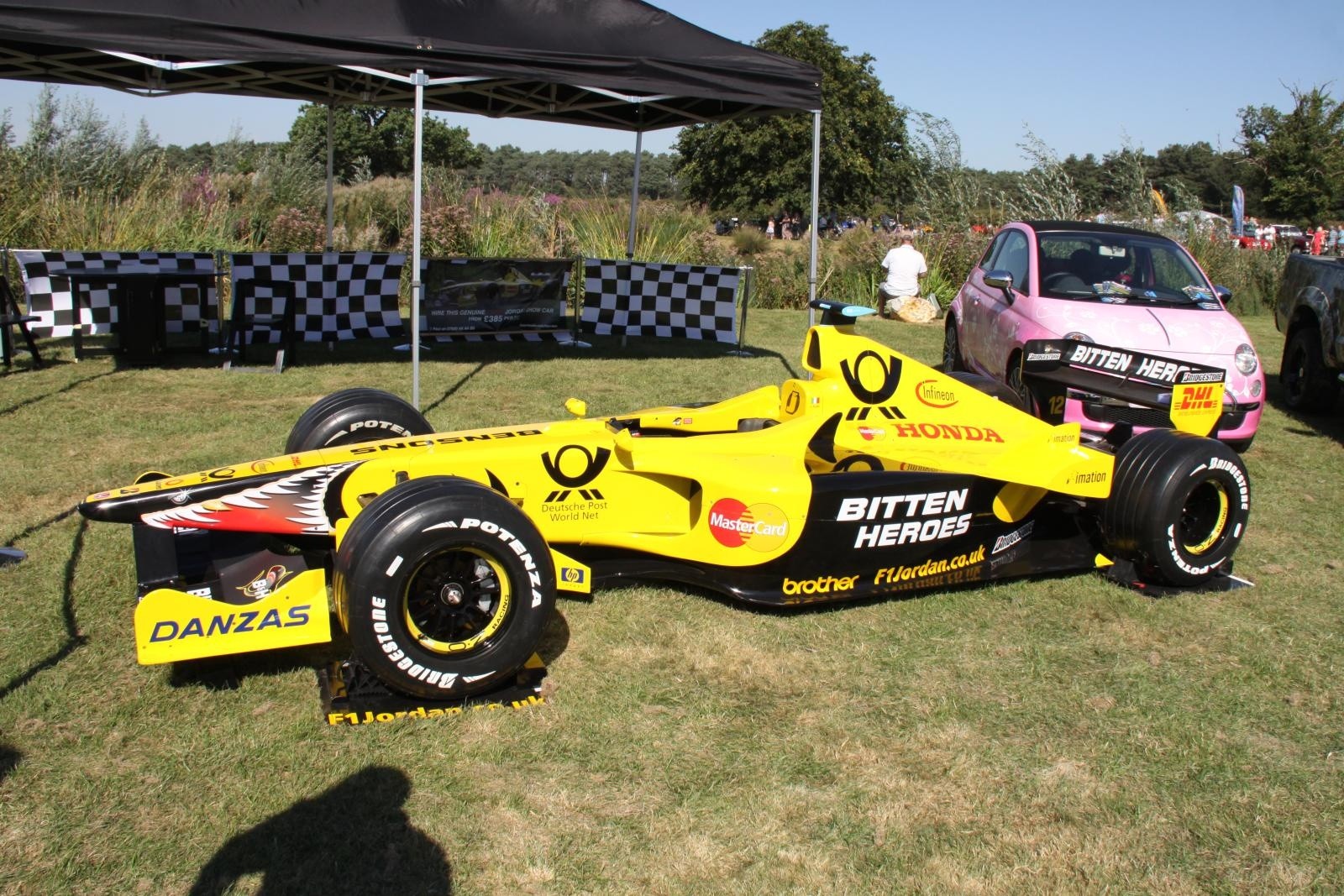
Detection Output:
[1273,225,1328,254]
[1230,224,1271,251]
[943,220,1265,452]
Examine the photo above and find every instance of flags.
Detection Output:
[1152,190,1169,215]
[1231,185,1244,237]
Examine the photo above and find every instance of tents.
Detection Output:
[1,0,831,406]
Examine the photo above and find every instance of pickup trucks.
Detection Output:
[1274,254,1344,414]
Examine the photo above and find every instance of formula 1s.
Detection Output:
[78,300,1254,728]
[433,267,546,305]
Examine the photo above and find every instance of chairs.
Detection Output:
[0,274,44,369]
[1012,246,1132,288]
[222,279,296,372]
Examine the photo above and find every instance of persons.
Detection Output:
[765,217,775,240]
[819,213,830,231]
[1306,224,1344,257]
[781,213,800,241]
[874,230,928,318]
[1242,215,1276,243]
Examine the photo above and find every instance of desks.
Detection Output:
[47,268,229,362]
